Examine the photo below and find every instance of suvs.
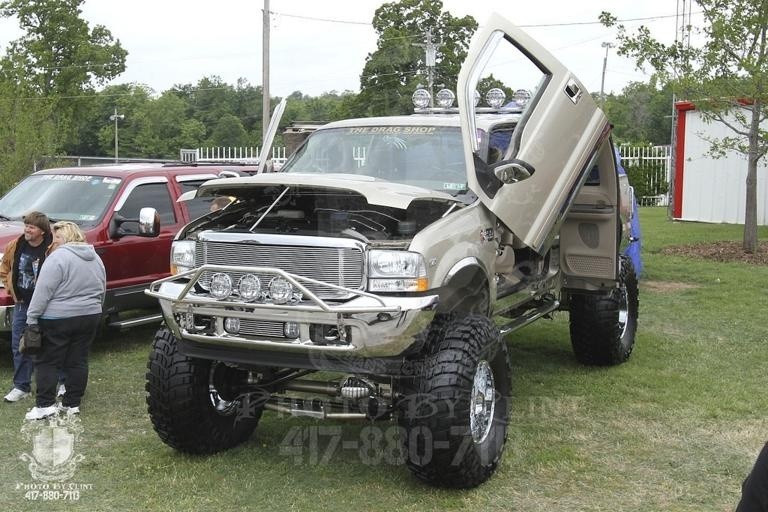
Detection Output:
[145,12,641,487]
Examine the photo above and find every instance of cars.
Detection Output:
[0,155,252,332]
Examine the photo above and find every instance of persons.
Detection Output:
[17,220,108,421]
[1,211,53,403]
[208,196,233,213]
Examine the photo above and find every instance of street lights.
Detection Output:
[110,108,125,164]
[601,41,617,110]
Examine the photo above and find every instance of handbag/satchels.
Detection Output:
[18,323,43,354]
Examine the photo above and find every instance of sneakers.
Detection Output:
[56,384,67,397]
[58,402,80,415]
[4,387,32,402]
[25,404,58,420]
